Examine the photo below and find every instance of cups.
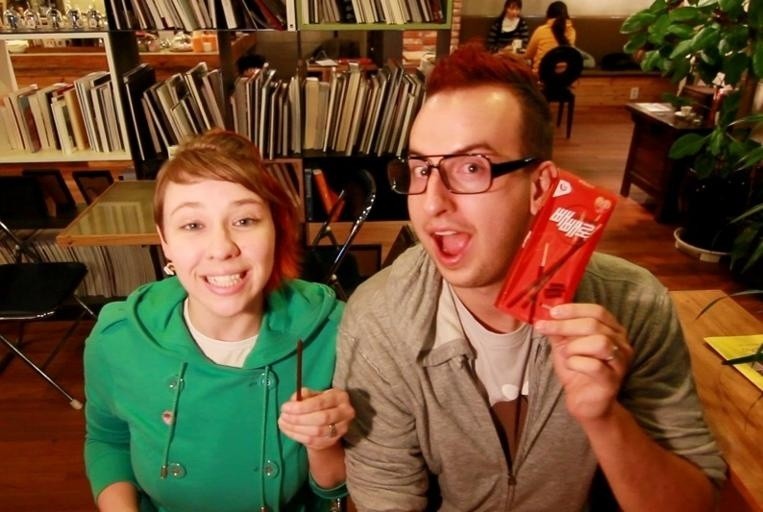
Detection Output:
[512,39,522,54]
[681,106,692,117]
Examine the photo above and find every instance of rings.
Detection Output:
[607,346,618,361]
[329,423,337,438]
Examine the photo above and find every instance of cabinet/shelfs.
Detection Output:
[620,77,757,224]
[1,0,462,242]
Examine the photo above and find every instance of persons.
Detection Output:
[523,0,579,91]
[487,0,530,54]
[332,37,729,512]
[84,126,356,512]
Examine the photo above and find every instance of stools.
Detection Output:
[0,223,98,411]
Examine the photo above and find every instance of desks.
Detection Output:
[660,290,762,510]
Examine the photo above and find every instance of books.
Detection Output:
[122,52,426,163]
[1,229,170,298]
[111,0,446,32]
[2,71,121,153]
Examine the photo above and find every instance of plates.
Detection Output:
[675,110,695,118]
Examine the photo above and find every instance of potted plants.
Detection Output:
[664,90,760,263]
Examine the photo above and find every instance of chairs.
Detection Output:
[536,47,583,139]
[298,167,377,301]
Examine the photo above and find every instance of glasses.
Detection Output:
[387,154,541,194]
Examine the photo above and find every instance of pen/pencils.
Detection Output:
[297,340,302,401]
